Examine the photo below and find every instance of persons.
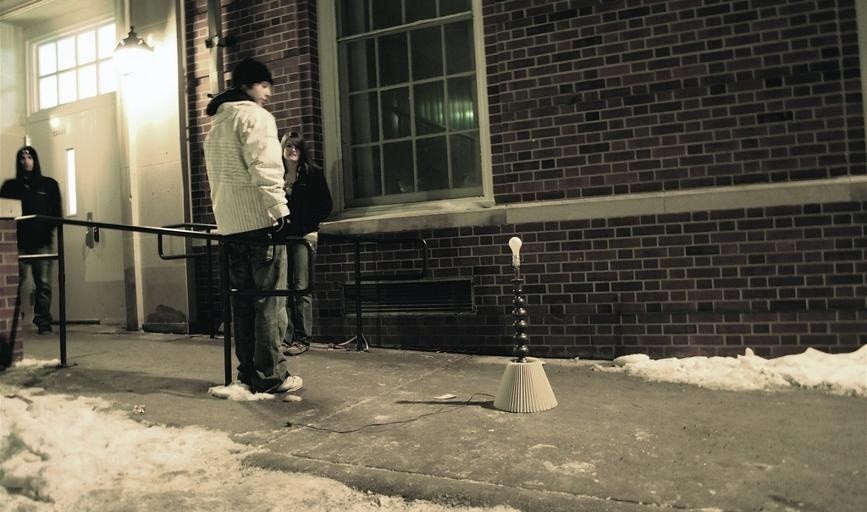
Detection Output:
[2,144,61,334]
[202,57,303,397]
[272,130,334,355]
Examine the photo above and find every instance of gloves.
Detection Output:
[265,216,288,241]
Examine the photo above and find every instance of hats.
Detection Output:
[232,57,272,86]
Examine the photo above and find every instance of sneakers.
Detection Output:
[39,325,52,335]
[278,342,288,354]
[284,341,310,355]
[252,375,303,395]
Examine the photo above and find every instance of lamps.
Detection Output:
[106,19,155,77]
